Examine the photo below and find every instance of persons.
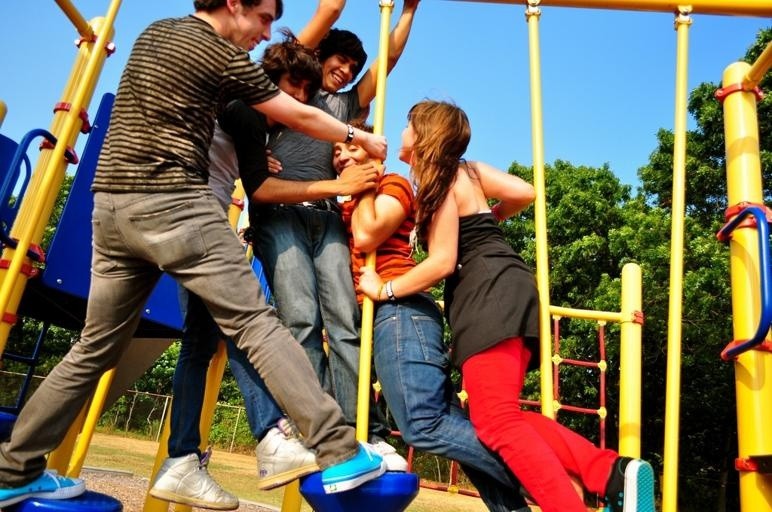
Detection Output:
[250,1,419,473]
[354,94,659,512]
[267,120,586,512]
[0,0,391,512]
[143,32,324,512]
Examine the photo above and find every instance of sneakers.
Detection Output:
[607,456,656,512]
[149,446,239,511]
[253,418,320,491]
[1,468,87,509]
[368,440,407,472]
[321,441,387,495]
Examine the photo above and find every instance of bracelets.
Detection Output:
[343,123,353,144]
[385,280,396,301]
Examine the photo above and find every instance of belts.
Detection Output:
[282,200,340,214]
